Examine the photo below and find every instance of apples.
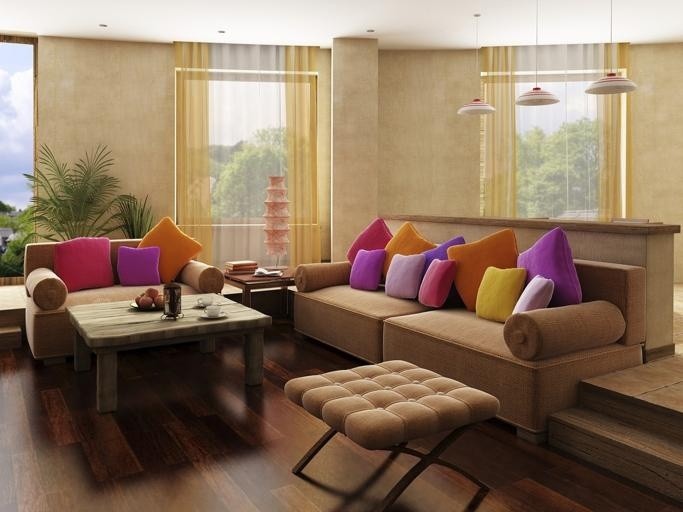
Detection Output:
[135,288,163,307]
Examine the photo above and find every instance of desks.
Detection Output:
[219,268,297,336]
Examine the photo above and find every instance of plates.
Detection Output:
[130,301,162,310]
[200,312,229,319]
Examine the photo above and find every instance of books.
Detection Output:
[225,260,257,274]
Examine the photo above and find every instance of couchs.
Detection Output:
[24,240,225,367]
[292,259,648,445]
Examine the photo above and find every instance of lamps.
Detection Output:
[456,0,638,116]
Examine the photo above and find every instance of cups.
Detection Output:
[198,296,214,306]
[204,306,221,316]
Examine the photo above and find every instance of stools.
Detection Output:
[284,359,500,511]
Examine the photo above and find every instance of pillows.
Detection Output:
[53,218,204,285]
[346,218,585,323]
